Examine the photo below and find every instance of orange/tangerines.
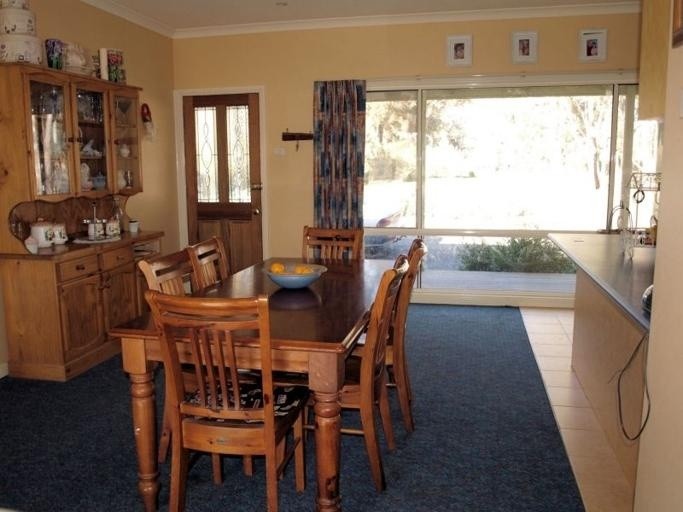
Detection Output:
[302,268,313,273]
[293,266,307,274]
[270,262,285,272]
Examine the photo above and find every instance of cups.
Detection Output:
[128,220,140,233]
[30,217,68,248]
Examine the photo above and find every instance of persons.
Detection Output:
[587,39,592,56]
[591,42,597,55]
[454,44,464,58]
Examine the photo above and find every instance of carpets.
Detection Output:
[1,300,588,512]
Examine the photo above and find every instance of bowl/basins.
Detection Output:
[262,264,329,288]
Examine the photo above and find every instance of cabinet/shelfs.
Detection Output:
[106,78,143,196]
[1,60,110,208]
[133,236,162,320]
[2,246,132,380]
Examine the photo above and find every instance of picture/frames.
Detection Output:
[445,28,609,66]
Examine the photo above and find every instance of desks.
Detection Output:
[105,256,394,511]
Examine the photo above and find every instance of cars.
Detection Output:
[363,184,412,258]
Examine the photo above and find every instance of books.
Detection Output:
[99,46,126,85]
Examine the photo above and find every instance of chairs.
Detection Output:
[187,236,231,290]
[285,254,396,494]
[349,238,428,433]
[145,290,307,512]
[302,225,366,261]
[140,247,203,317]
